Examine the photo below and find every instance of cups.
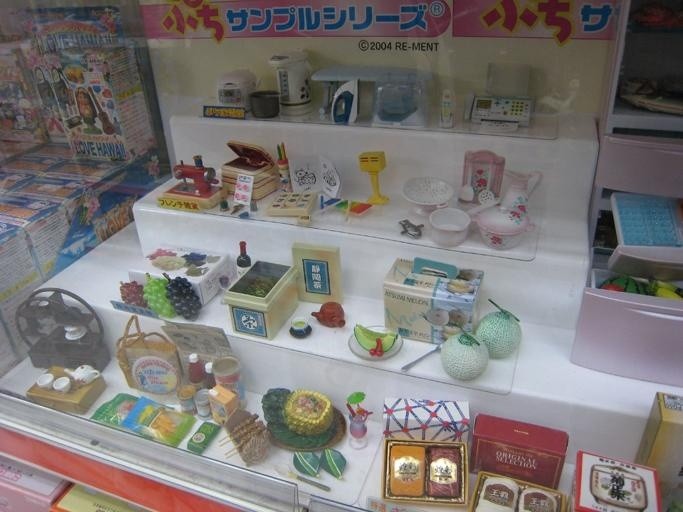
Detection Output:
[35,371,71,394]
[61,326,83,337]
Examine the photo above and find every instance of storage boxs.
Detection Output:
[0,1,159,378]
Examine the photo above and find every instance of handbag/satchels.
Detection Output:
[116,315,182,394]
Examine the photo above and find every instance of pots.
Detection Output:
[470,208,536,250]
[428,208,472,249]
[248,89,281,118]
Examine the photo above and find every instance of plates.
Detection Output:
[401,176,455,207]
[346,322,404,362]
[64,327,87,341]
[264,404,348,453]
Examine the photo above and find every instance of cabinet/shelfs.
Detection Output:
[571,112,683,389]
[128,95,592,336]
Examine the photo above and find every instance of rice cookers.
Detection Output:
[215,69,258,113]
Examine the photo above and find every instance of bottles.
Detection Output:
[236,240,252,280]
[187,353,213,389]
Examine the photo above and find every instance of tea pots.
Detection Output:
[500,166,544,211]
[63,364,101,387]
